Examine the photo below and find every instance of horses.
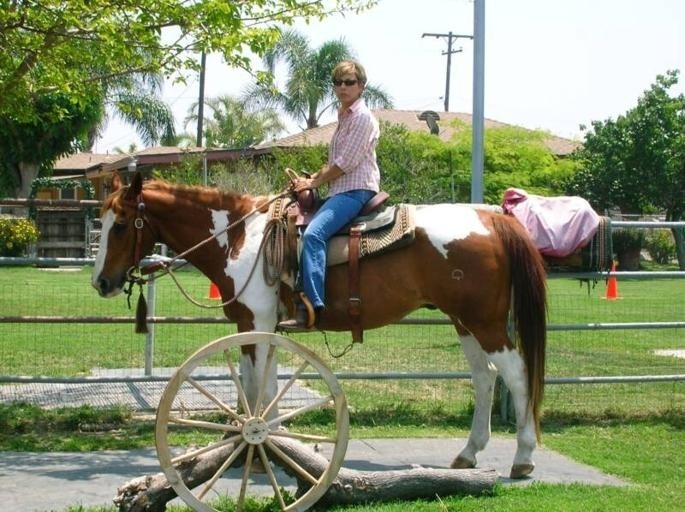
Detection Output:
[89,172,549,481]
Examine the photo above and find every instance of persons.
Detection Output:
[279,60,380,330]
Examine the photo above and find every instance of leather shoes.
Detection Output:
[279,310,320,328]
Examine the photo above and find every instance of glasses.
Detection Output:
[332,79,356,86]
